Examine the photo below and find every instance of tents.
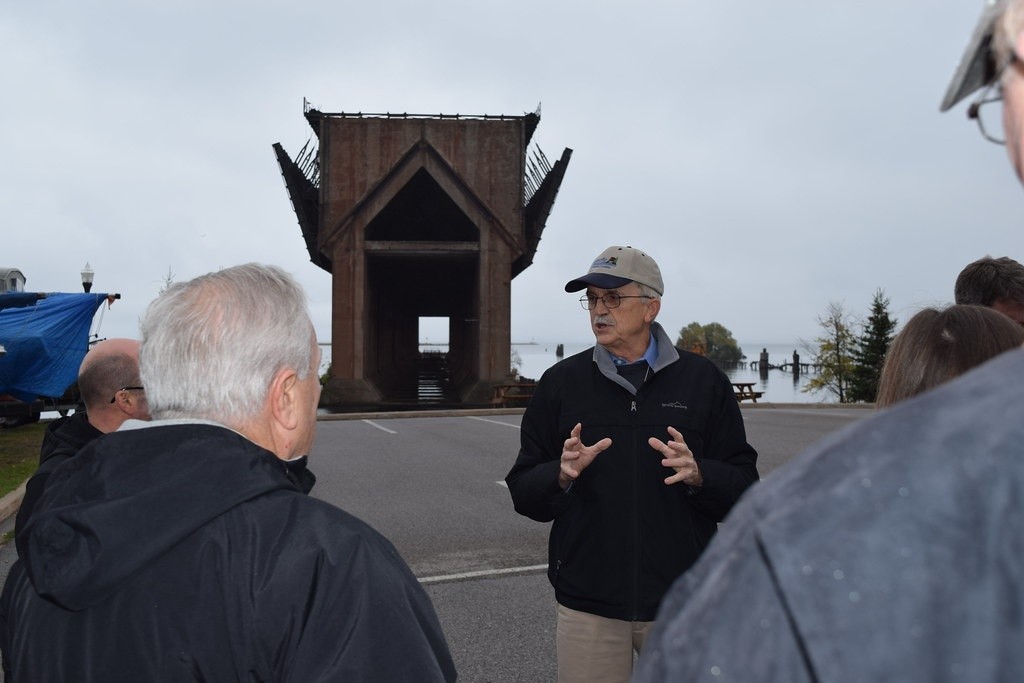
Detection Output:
[0,292,47,312]
[0,292,122,406]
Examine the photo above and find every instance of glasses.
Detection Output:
[965,50,1021,147]
[580,292,653,311]
[109,384,146,404]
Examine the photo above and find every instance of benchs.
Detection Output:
[732,382,765,404]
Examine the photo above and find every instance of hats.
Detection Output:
[938,1,1023,112]
[563,244,665,297]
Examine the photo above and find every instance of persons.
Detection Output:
[504,244,763,682]
[0,261,459,683]
[952,254,1024,328]
[14,337,156,561]
[870,300,1023,415]
[626,0,1023,683]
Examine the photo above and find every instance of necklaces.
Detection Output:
[643,365,650,383]
